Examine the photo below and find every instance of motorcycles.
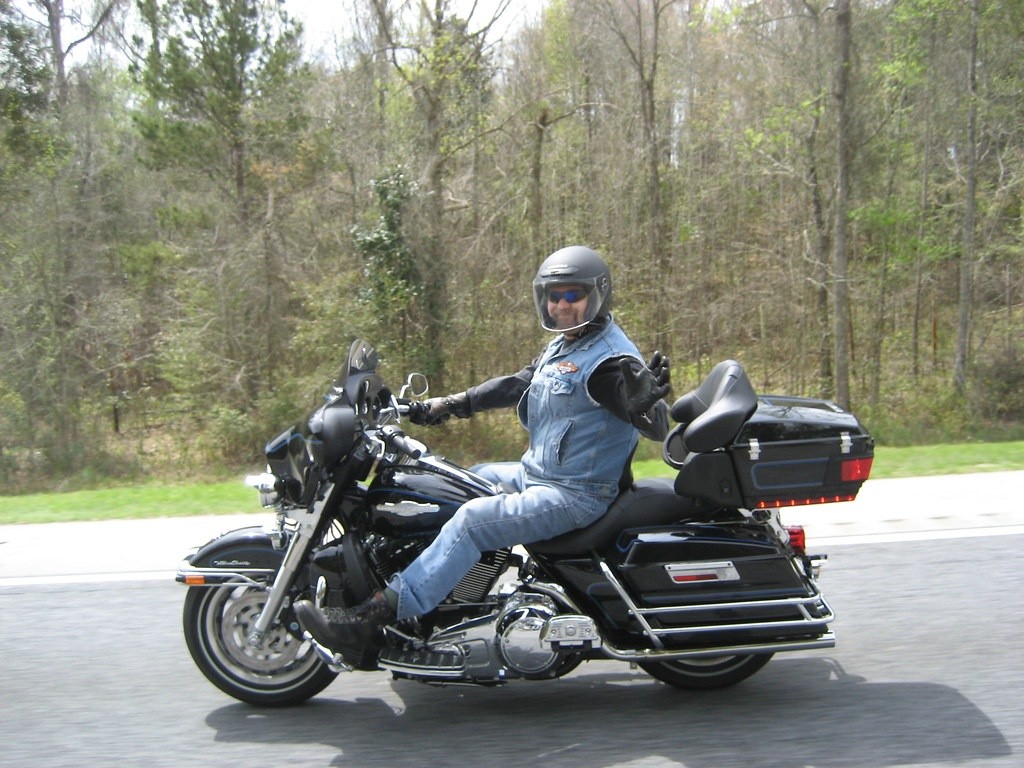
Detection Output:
[173,342,876,709]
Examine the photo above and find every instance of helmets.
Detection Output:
[532,245,612,331]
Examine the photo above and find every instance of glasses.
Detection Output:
[546,288,589,304]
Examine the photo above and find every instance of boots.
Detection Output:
[293,588,397,670]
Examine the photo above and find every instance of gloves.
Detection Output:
[618,350,671,429]
[408,396,452,426]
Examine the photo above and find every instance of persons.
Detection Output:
[292,246,671,668]
